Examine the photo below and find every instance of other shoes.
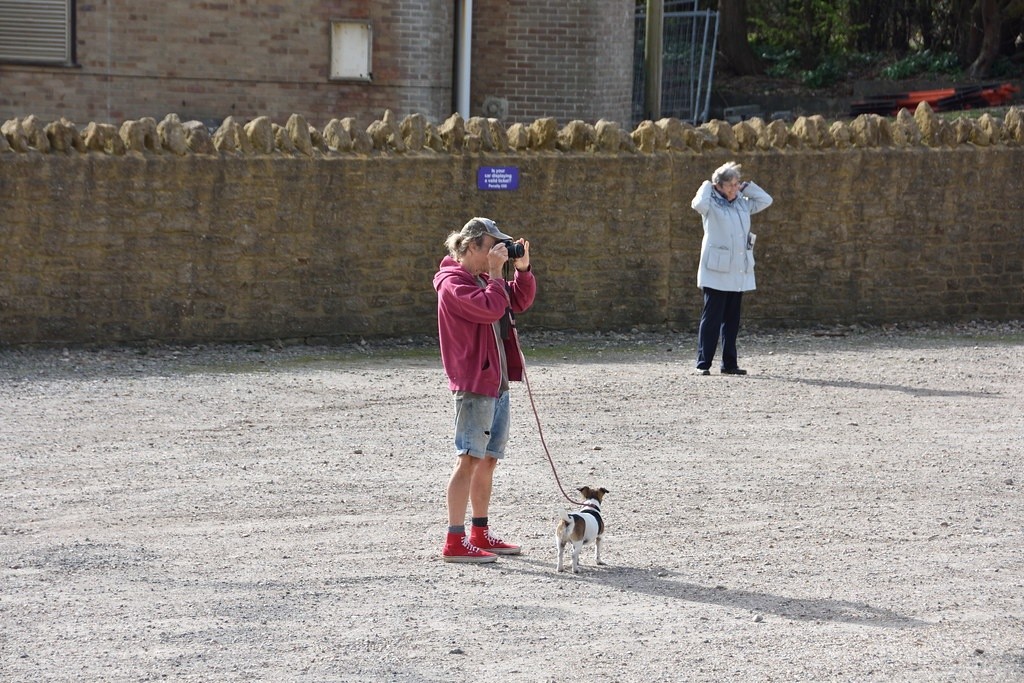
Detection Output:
[698,366,710,375]
[721,367,747,374]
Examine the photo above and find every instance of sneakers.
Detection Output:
[468,525,521,555]
[442,531,498,563]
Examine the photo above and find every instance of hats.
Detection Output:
[461,215,514,242]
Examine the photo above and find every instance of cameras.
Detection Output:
[492,239,524,258]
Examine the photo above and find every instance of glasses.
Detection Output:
[721,182,740,189]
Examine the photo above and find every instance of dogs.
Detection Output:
[556,486,609,574]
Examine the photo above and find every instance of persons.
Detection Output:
[692,161,773,376]
[433,217,536,563]
